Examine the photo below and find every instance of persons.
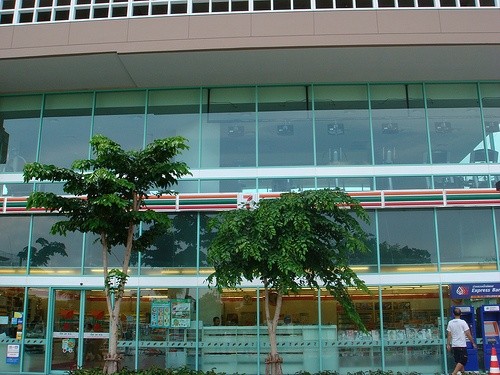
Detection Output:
[446,308,477,375]
[0,309,295,356]
